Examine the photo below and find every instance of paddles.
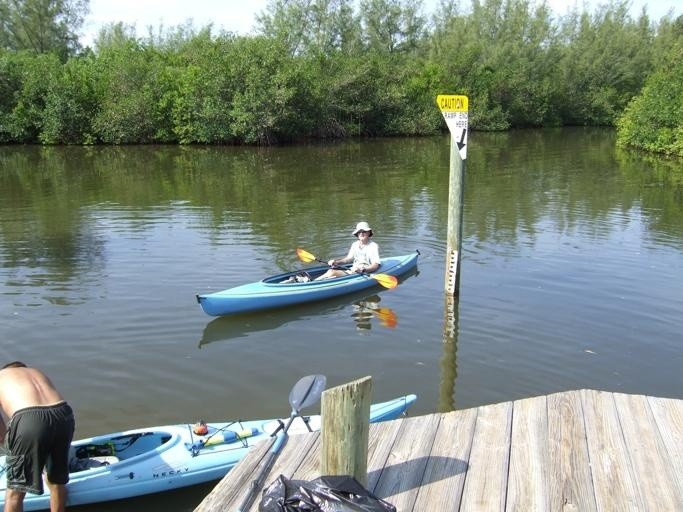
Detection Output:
[354,302,396,328]
[236,374,326,512]
[297,248,398,289]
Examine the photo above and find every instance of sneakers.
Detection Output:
[296,276,311,283]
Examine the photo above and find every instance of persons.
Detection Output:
[0,361,75,512]
[316,222,381,279]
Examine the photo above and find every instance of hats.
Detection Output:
[352,222,373,237]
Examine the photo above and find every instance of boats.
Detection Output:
[195,250,422,317]
[0,393,418,512]
[197,273,420,351]
